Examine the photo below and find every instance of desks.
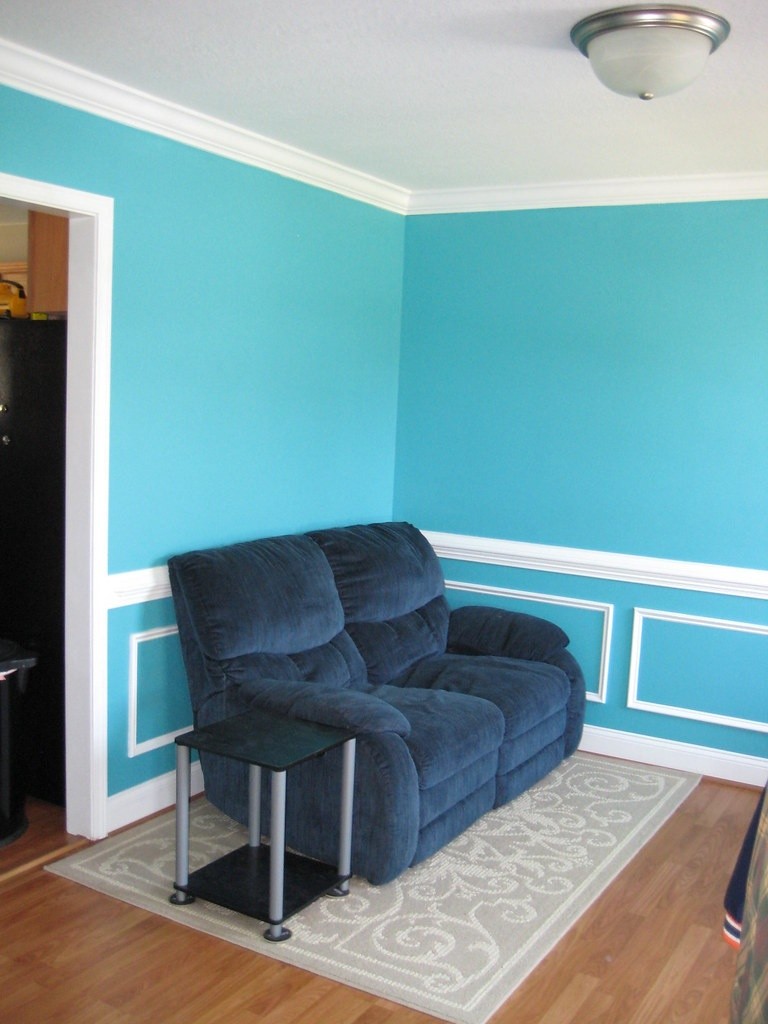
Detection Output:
[0,637,39,848]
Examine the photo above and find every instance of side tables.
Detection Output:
[169,710,359,942]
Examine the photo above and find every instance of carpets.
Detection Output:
[44,754,703,1024]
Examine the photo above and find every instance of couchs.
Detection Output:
[166,521,587,885]
